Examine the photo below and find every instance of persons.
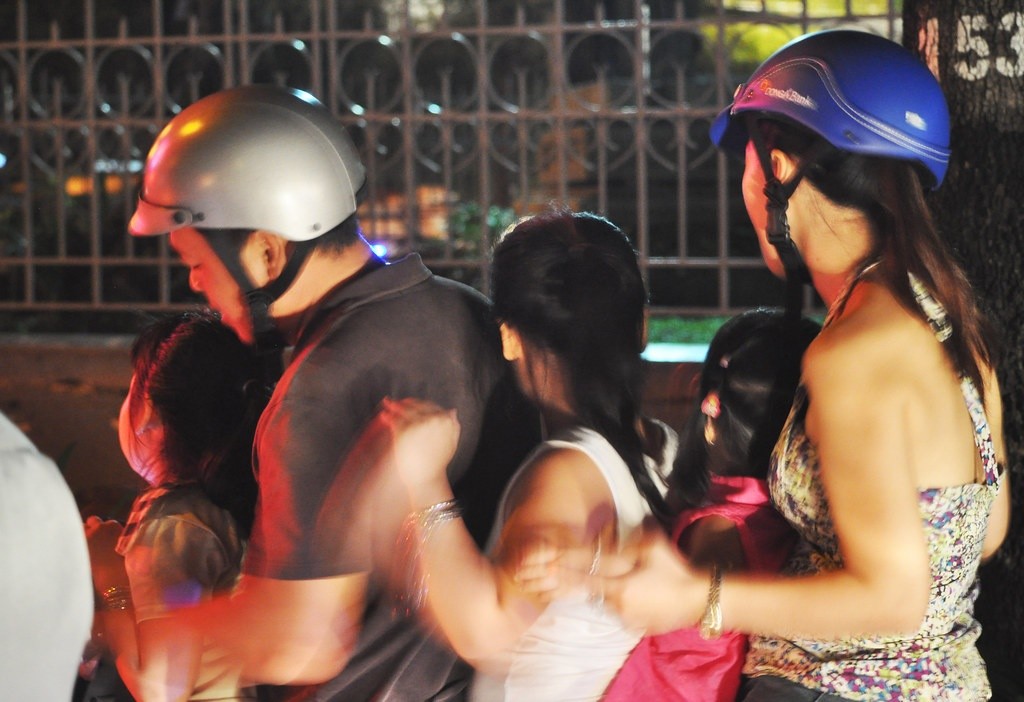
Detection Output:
[76,82,541,702]
[598,307,822,702]
[376,212,711,702]
[83,305,285,702]
[545,31,1012,702]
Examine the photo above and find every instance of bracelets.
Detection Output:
[693,565,723,642]
[103,586,133,611]
[419,500,466,519]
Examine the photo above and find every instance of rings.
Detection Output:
[590,547,600,574]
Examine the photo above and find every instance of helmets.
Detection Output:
[128,85,366,242]
[709,29,950,189]
